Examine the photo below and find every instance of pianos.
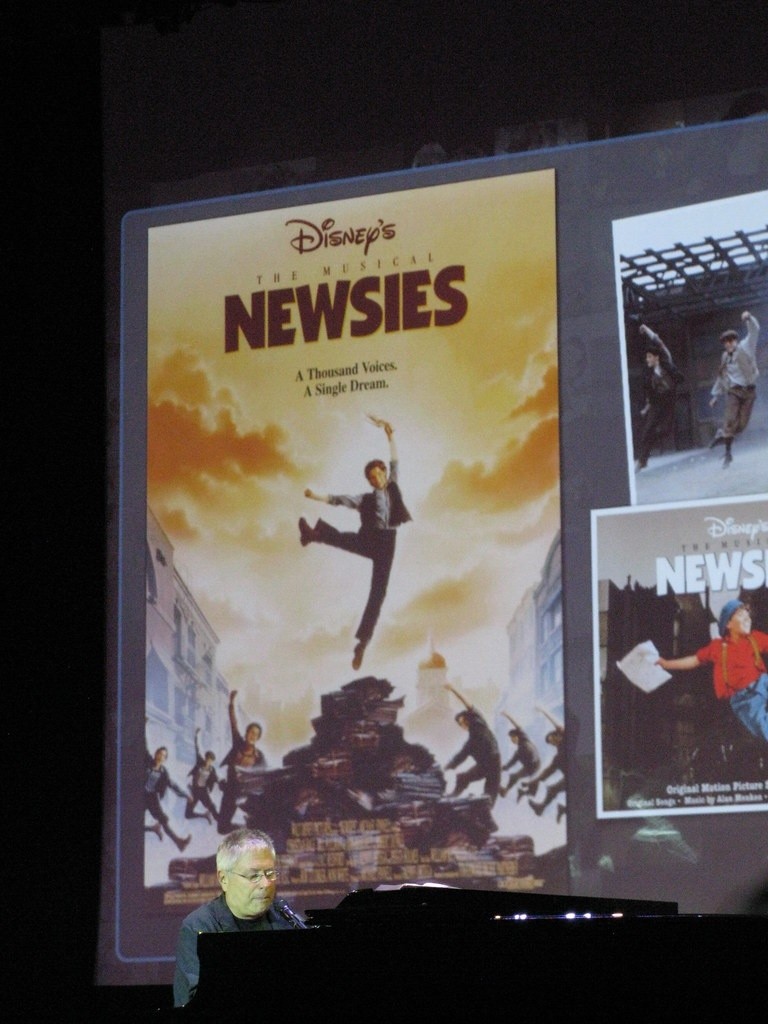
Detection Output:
[172,884,768,1023]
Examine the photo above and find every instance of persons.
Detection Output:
[184,727,225,826]
[145,718,195,851]
[443,680,501,808]
[655,599,768,760]
[632,324,685,473]
[216,690,263,833]
[706,311,759,471]
[497,709,540,796]
[298,409,412,669]
[174,829,296,1005]
[517,708,567,826]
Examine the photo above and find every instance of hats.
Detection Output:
[720,330,739,339]
[718,598,744,637]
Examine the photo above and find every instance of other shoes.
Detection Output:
[352,643,363,669]
[516,789,524,803]
[175,834,193,852]
[298,517,310,547]
[708,437,720,448]
[153,823,164,841]
[721,454,732,469]
[506,773,517,789]
[204,811,213,825]
[556,803,564,823]
[528,799,544,816]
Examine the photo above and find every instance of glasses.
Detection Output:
[232,870,280,883]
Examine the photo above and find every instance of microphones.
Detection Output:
[272,897,306,929]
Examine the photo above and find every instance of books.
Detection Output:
[615,640,672,694]
